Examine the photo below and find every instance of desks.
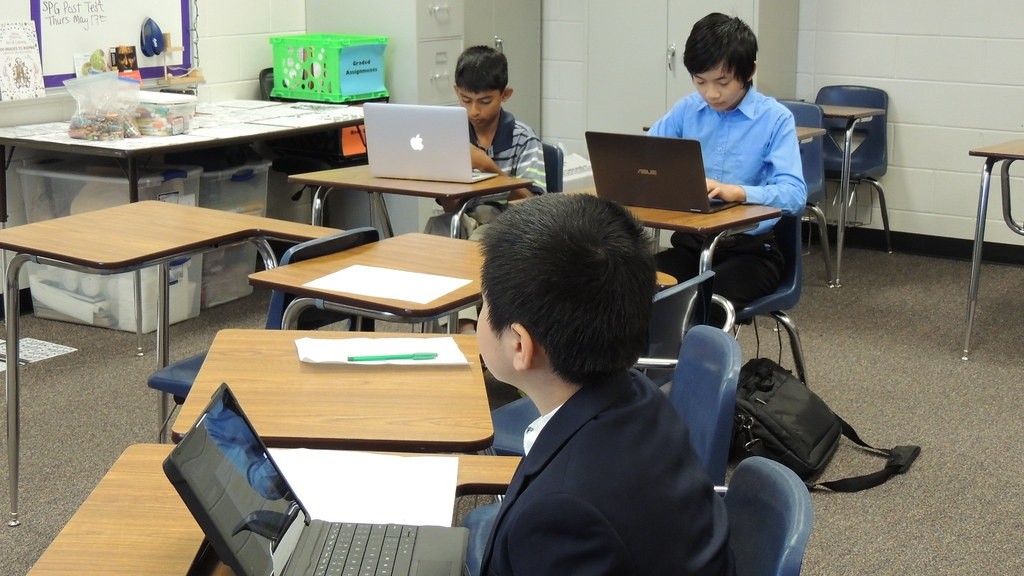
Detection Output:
[24,442,522,576]
[816,104,886,289]
[171,328,493,451]
[509,188,783,329]
[242,232,484,331]
[960,139,1024,362]
[0,99,369,357]
[0,199,345,526]
[288,166,534,331]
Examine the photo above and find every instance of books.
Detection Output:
[110,45,138,72]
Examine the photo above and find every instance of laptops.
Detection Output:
[363,103,499,183]
[585,131,742,214]
[162,383,468,575]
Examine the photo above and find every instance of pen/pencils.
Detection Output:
[348,353,438,361]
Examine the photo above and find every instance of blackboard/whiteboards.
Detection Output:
[30,0,191,88]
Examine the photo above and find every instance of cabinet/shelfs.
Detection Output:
[304,1,541,132]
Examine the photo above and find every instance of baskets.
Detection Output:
[270,33,390,103]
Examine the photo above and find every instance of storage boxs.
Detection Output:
[271,32,391,100]
[201,241,258,308]
[28,252,203,333]
[199,155,273,217]
[17,160,203,223]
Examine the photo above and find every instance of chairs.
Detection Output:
[148,82,893,576]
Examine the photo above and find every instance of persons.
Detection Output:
[647,12,807,312]
[424,45,547,240]
[476,192,736,576]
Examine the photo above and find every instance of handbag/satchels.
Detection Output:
[728,356,921,493]
